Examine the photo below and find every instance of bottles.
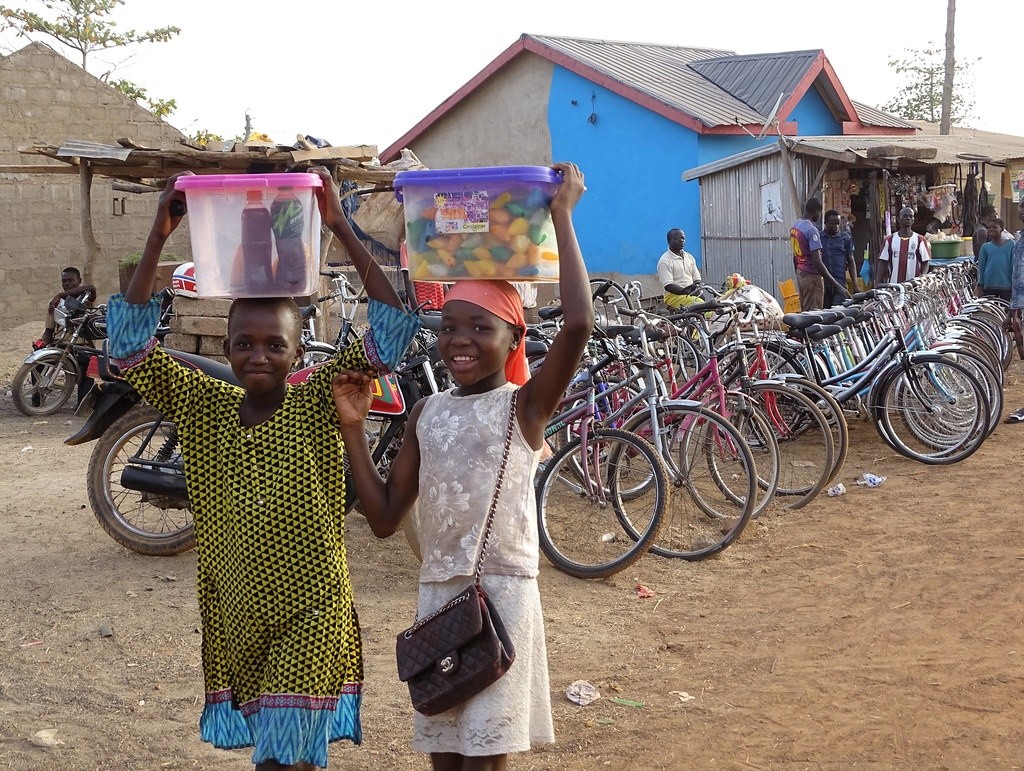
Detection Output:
[241,190,274,294]
[270,185,307,293]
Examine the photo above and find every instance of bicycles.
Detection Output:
[291,260,1014,579]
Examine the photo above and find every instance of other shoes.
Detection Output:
[1004,407,1024,423]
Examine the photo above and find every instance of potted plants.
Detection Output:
[118,249,187,294]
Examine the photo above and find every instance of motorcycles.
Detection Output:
[11,292,173,418]
[64,300,431,564]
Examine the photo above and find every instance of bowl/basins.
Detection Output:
[931,240,964,258]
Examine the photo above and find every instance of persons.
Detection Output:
[397,238,450,314]
[32,268,98,410]
[102,168,420,771]
[508,281,540,324]
[656,228,713,339]
[972,206,999,261]
[330,162,593,770]
[876,207,931,286]
[975,218,1015,305]
[789,199,852,311]
[1001,195,1023,423]
[820,210,860,309]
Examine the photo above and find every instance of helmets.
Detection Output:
[172,264,221,300]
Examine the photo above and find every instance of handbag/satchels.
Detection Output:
[395,583,516,717]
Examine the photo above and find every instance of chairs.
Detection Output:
[778,279,800,313]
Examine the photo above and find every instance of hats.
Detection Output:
[442,279,528,386]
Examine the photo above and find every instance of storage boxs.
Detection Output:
[390,166,562,282]
[175,173,323,299]
